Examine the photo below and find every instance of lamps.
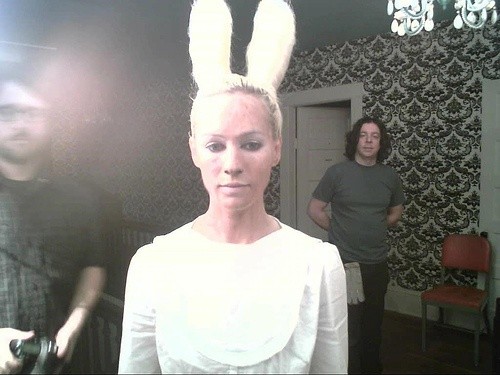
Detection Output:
[387,0,497,35]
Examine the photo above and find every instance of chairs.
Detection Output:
[421,233,493,366]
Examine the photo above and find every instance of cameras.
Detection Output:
[11,337,60,375]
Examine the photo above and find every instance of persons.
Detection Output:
[118,74,349,375]
[0,81,109,374]
[306,116,405,375]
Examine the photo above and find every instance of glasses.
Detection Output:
[0,108,47,122]
